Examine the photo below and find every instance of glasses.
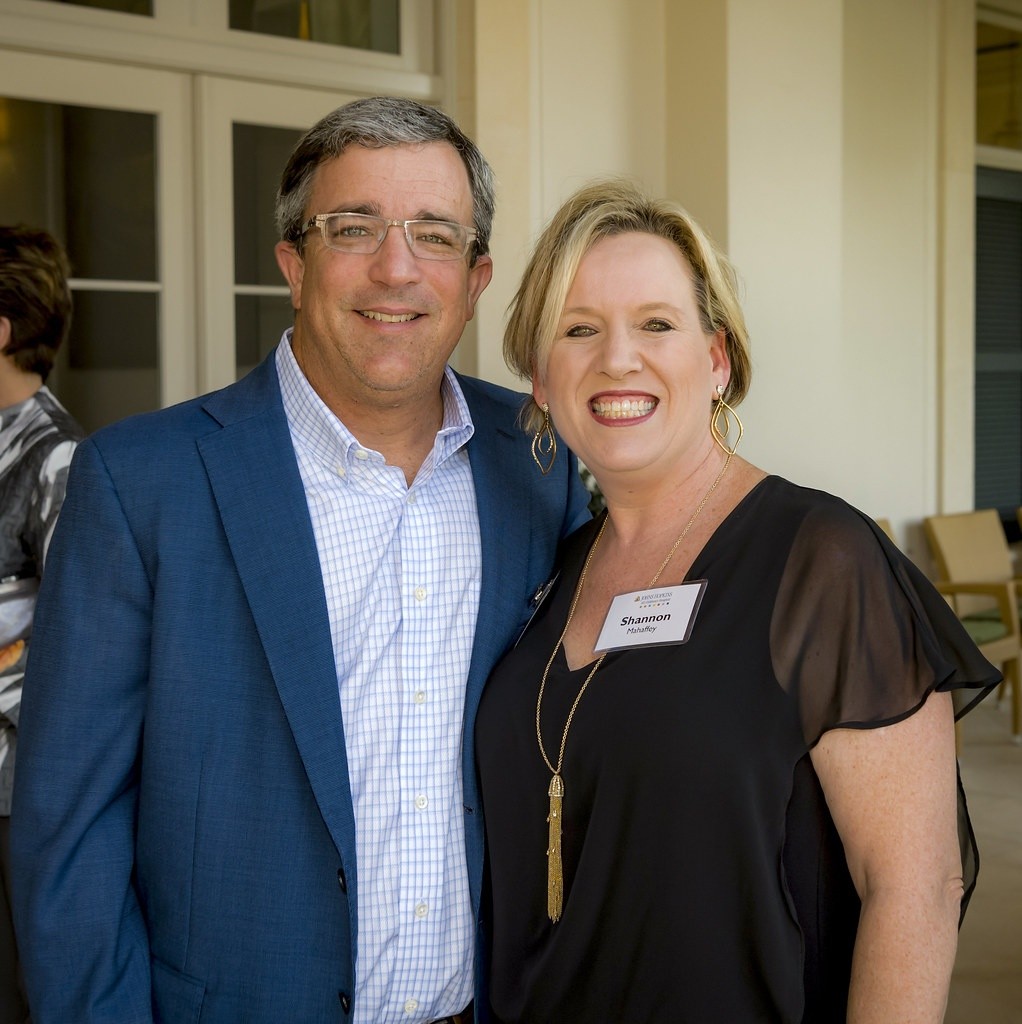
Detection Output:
[297,212,483,261]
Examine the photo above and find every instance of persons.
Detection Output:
[20,97,599,1022]
[0,224,85,1024]
[477,184,1000,1021]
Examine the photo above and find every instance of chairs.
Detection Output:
[873,519,1022,745]
[926,508,1022,702]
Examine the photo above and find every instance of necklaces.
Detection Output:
[533,453,743,928]
